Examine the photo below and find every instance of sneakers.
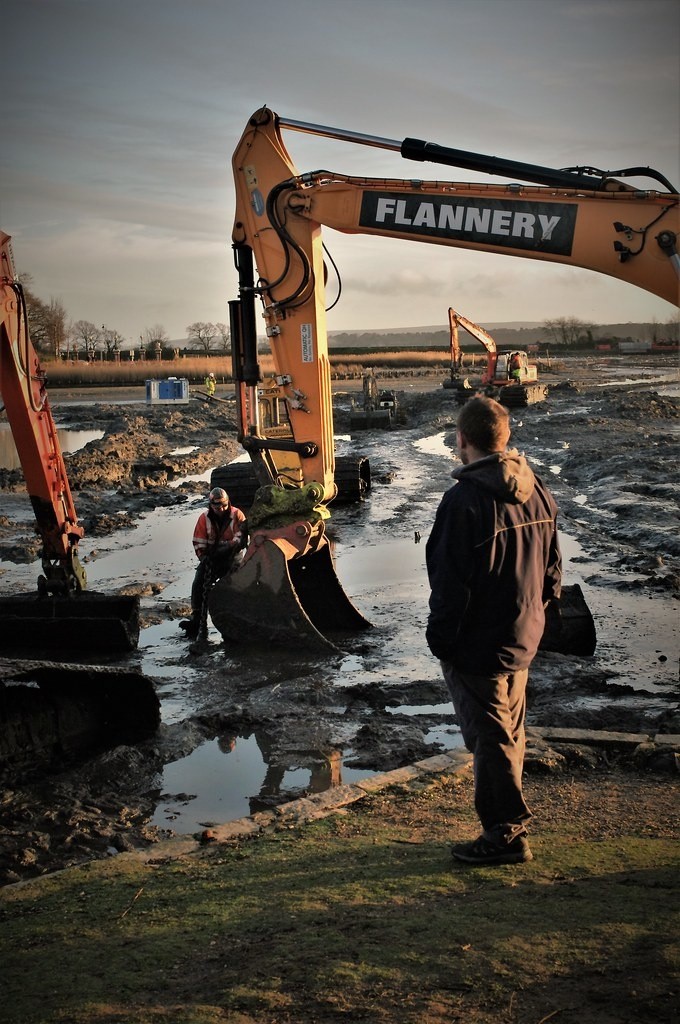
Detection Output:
[452,835,533,864]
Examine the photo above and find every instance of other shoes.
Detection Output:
[179,615,198,632]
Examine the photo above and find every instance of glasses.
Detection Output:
[214,503,227,509]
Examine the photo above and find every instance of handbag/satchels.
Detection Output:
[535,583,597,657]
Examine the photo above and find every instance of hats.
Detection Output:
[209,488,229,505]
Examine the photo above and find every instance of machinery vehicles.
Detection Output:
[349,373,399,428]
[209,372,371,508]
[0,226,141,657]
[443,306,548,407]
[201,107,680,667]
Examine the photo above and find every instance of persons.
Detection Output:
[510,352,522,385]
[205,373,216,404]
[179,488,250,656]
[425,395,564,866]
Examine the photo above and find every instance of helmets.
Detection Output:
[209,372,215,377]
